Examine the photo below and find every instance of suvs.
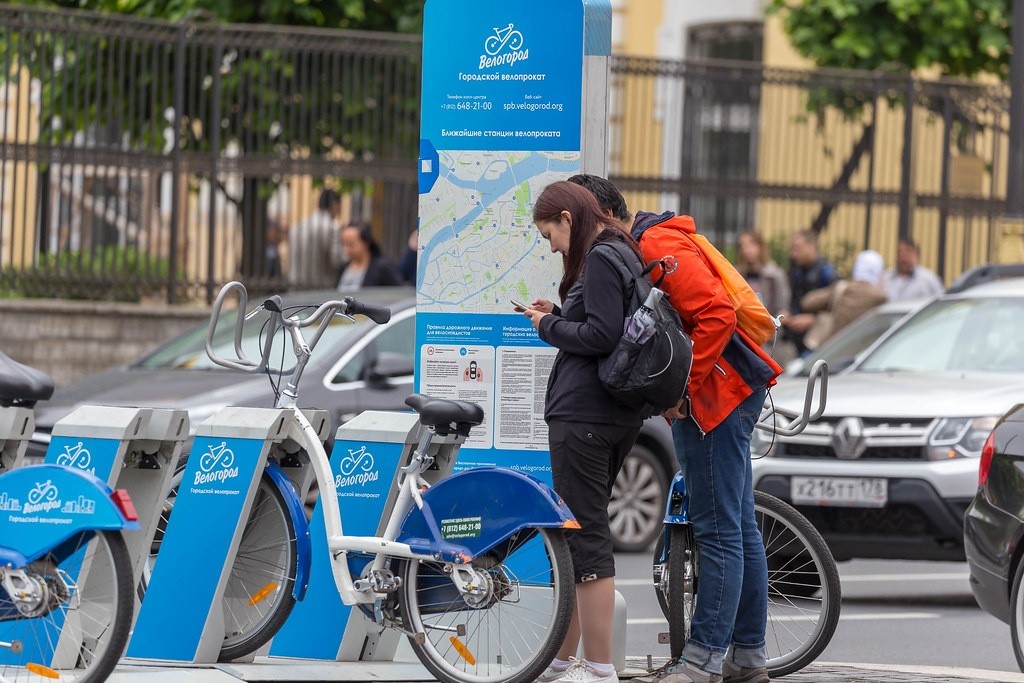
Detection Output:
[751,263,1024,597]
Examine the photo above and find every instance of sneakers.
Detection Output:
[723,660,770,683]
[549,656,619,683]
[533,663,570,683]
[628,657,724,683]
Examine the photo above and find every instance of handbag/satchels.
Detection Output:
[802,279,850,351]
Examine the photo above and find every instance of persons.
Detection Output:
[567,174,784,683]
[335,219,400,288]
[288,189,346,290]
[240,217,289,290]
[514,180,693,683]
[730,229,946,368]
[400,227,418,287]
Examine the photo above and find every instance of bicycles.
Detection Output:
[137,282,582,683]
[652,314,842,678]
[0,350,143,683]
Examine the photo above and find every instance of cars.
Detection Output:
[25,286,679,553]
[963,404,1024,673]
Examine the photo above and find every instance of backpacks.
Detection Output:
[587,242,694,416]
[692,234,776,347]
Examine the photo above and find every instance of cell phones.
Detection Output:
[511,299,531,311]
